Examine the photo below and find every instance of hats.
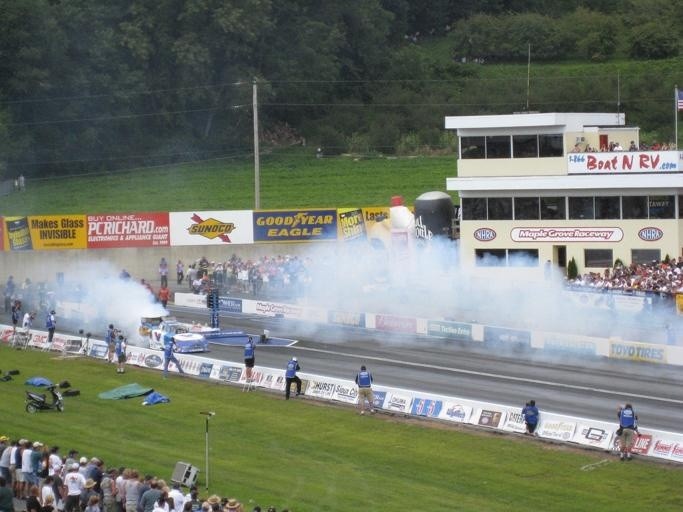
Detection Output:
[83,479,97,488]
[0,435,44,447]
[50,445,101,471]
[208,494,220,504]
[226,499,240,509]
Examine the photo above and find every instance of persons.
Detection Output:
[617,405,638,460]
[521,400,539,433]
[0,435,291,512]
[119,254,314,309]
[115,336,126,373]
[563,260,683,295]
[571,140,676,152]
[6,276,57,342]
[285,357,304,400]
[163,337,184,376]
[244,336,256,383]
[106,324,118,363]
[355,366,375,414]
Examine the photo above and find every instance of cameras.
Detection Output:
[114,328,122,334]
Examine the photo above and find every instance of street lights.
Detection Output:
[200,411,216,490]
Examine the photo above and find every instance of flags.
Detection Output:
[678,90,683,111]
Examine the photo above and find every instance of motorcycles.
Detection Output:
[23,382,64,413]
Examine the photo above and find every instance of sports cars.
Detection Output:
[139,316,207,353]
